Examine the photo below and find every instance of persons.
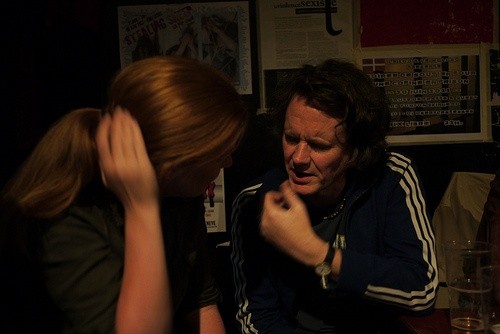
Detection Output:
[0,57,227,334]
[230,56,440,334]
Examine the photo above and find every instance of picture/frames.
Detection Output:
[112,0,260,107]
[354,44,493,145]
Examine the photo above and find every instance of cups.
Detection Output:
[446,241,497,334]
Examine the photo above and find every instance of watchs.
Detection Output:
[315,244,336,276]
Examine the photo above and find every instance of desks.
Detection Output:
[399,305,500,334]
